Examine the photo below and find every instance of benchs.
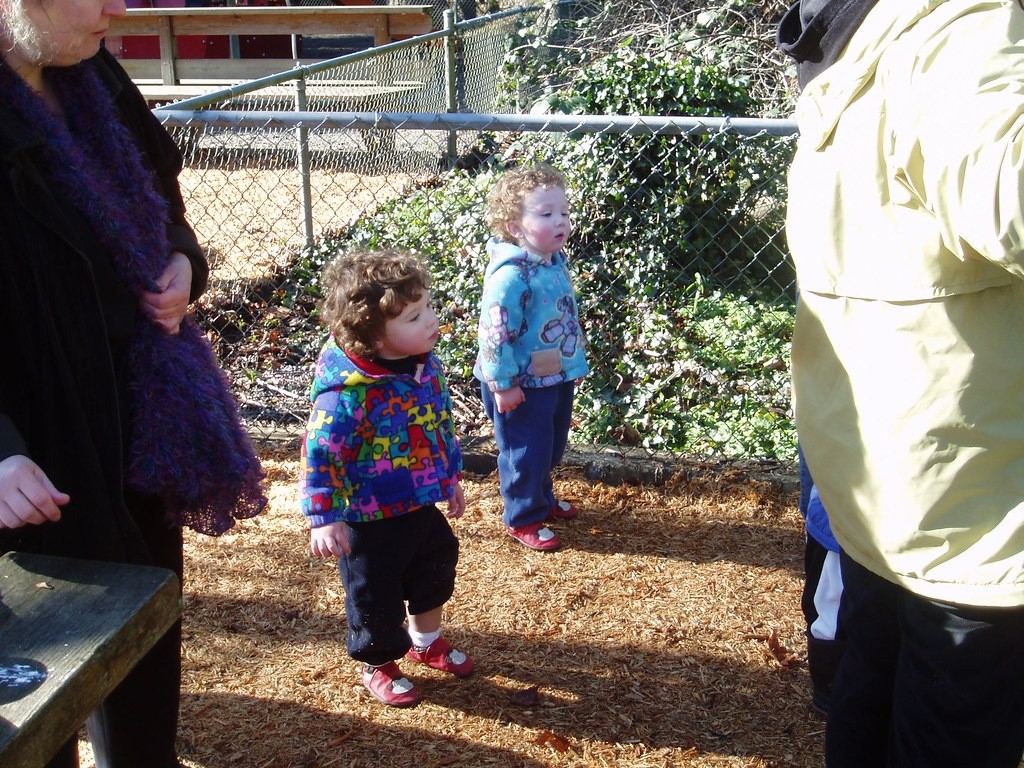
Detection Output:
[105,5,435,166]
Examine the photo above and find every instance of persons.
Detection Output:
[0,1,269,768]
[471,164,589,551]
[299,250,476,708]
[775,0,1023,768]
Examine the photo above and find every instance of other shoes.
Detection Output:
[363,661,418,705]
[549,500,578,518]
[507,521,561,550]
[406,636,474,675]
